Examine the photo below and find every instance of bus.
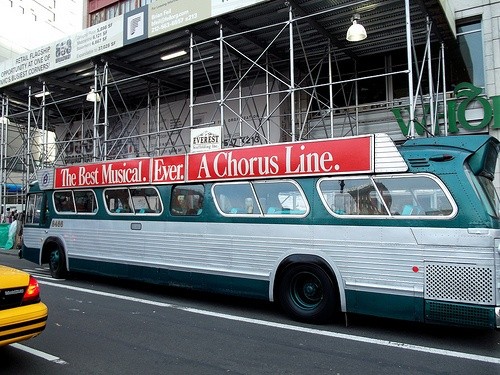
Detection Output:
[15,135,500,332]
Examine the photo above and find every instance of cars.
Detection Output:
[0,263,48,348]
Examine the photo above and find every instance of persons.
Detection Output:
[7,209,48,243]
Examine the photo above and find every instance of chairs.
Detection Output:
[87,204,428,215]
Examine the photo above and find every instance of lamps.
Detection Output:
[160,49,188,61]
[86,84,101,102]
[33,91,52,97]
[345,14,367,42]
